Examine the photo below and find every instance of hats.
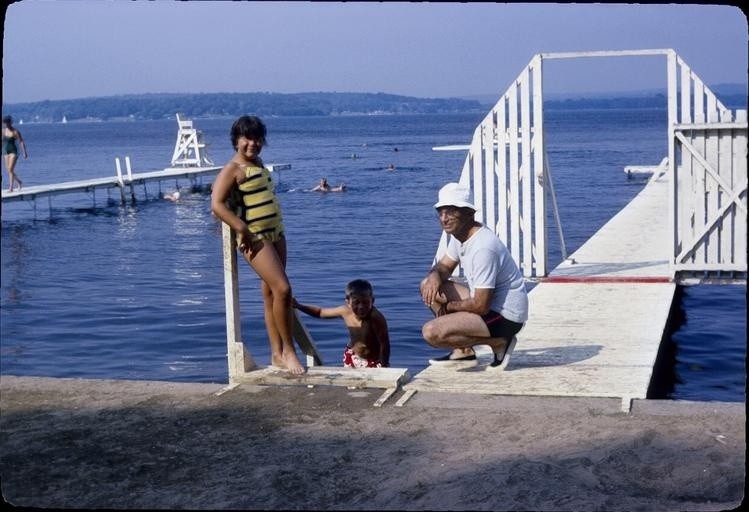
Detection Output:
[431,181,479,215]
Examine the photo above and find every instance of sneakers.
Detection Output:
[485,335,517,374]
[427,350,479,368]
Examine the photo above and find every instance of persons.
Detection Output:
[210,114,307,376]
[311,176,347,193]
[1,114,29,193]
[291,279,393,369]
[419,180,531,373]
[162,192,184,202]
